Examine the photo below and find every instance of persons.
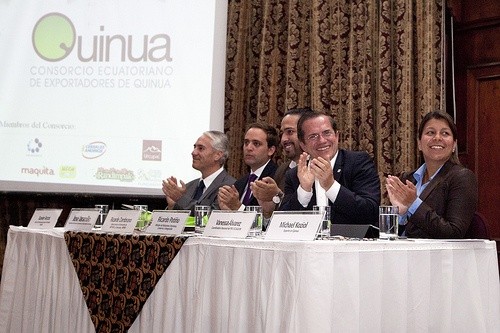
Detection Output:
[216,123,279,212]
[249,105,313,232]
[384,109,479,241]
[162,129,239,217]
[282,110,381,227]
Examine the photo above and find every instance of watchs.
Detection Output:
[271,190,284,205]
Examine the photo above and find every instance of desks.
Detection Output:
[0,226,500,333]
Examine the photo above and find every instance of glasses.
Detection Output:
[304,130,335,142]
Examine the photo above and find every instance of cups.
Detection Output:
[246,206,263,238]
[379,206,399,240]
[134,205,148,229]
[95,205,108,227]
[195,205,211,234]
[313,206,331,240]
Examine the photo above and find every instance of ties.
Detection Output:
[242,173,258,205]
[193,179,205,200]
